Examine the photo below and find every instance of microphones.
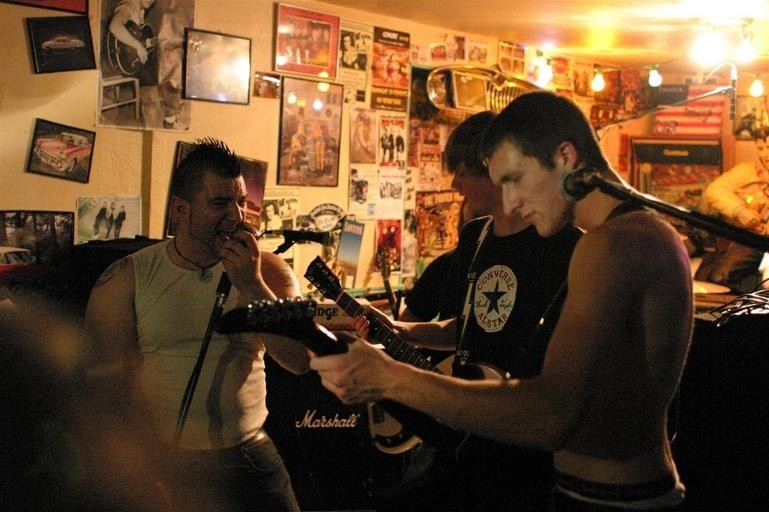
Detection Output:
[266,229,336,246]
[559,167,597,202]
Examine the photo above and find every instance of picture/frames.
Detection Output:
[182,27,253,108]
[277,76,345,187]
[0,0,91,15]
[25,116,97,184]
[25,13,97,74]
[0,209,75,267]
[273,2,341,80]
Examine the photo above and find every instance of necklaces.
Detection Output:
[174,237,220,283]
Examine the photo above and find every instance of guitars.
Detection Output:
[214,257,554,499]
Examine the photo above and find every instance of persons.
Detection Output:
[341,34,361,70]
[109,0,189,130]
[734,108,757,137]
[83,137,313,512]
[261,202,283,231]
[704,126,769,285]
[284,27,330,64]
[309,92,694,511]
[93,201,126,240]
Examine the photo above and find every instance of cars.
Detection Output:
[40,36,85,53]
[0,246,33,273]
[33,132,93,175]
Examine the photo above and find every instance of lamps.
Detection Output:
[533,49,764,97]
